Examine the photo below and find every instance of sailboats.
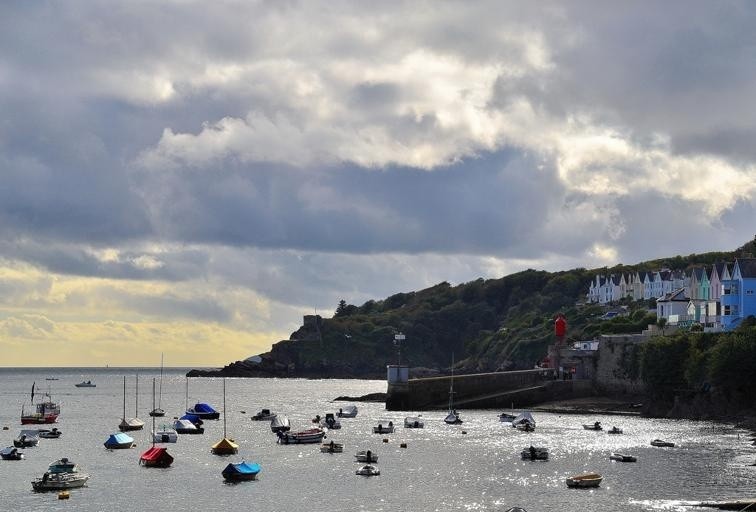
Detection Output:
[444,352,462,425]
[211,378,238,450]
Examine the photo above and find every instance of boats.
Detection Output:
[354,449,378,462]
[499,402,537,432]
[221,461,261,479]
[250,404,357,453]
[373,420,395,433]
[0,352,220,468]
[583,420,602,430]
[608,428,623,433]
[404,416,424,428]
[355,464,380,475]
[609,452,638,463]
[650,437,676,448]
[565,472,603,487]
[520,447,548,459]
[30,471,92,491]
[48,458,78,471]
